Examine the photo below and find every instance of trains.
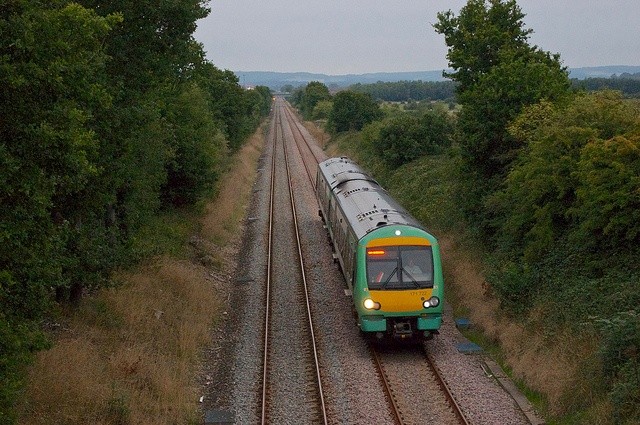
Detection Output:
[315,156,444,346]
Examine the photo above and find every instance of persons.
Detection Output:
[403,259,422,273]
